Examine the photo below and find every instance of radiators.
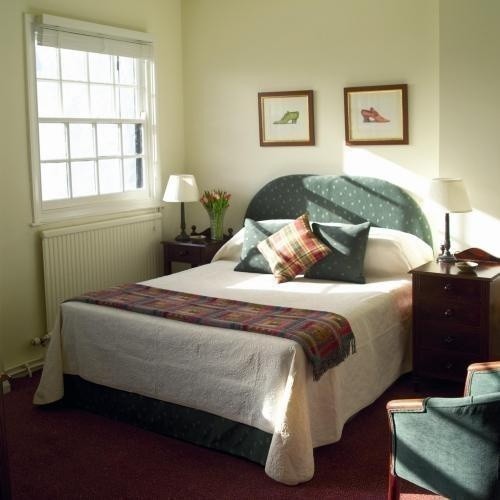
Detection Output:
[31,213,163,345]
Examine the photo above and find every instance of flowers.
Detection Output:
[197,186,232,241]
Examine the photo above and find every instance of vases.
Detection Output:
[208,209,225,240]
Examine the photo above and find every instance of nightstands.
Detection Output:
[159,228,234,275]
[407,248,500,395]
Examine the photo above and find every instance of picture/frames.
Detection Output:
[258,90,317,147]
[344,83,410,146]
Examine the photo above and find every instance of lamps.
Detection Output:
[427,178,473,263]
[162,174,202,240]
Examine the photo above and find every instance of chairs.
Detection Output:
[384,362,499,500]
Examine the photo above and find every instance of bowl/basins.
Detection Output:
[189,235,206,243]
[455,262,478,273]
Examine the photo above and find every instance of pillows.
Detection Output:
[256,214,332,283]
[234,218,288,274]
[303,221,370,284]
[210,219,295,263]
[363,227,434,277]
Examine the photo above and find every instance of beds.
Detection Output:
[28,175,434,484]
